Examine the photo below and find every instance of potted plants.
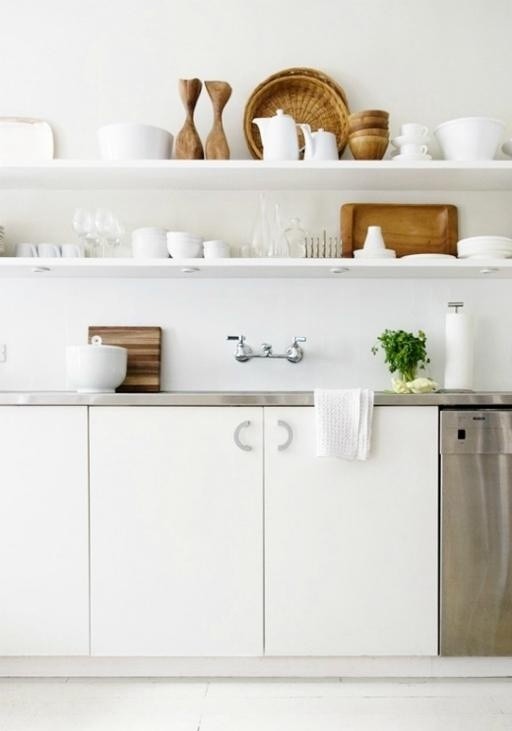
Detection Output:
[372,328,437,394]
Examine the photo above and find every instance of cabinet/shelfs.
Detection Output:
[88,407,439,681]
[0,159,512,281]
[1,406,88,678]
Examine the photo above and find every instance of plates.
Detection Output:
[351,249,396,259]
[0,115,55,164]
[392,137,436,144]
[392,156,432,162]
[400,236,509,259]
[0,224,5,256]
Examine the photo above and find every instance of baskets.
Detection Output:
[244,66,350,160]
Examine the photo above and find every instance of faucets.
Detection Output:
[285,337,306,363]
[225,335,251,362]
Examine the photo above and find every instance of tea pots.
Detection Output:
[300,123,339,161]
[252,109,310,162]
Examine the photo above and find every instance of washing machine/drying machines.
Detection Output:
[439,409,511,656]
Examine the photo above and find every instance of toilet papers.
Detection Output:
[444,313,476,389]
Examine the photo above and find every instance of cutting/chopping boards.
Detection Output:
[87,325,161,393]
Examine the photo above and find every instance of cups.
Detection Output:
[401,146,427,156]
[16,243,82,258]
[399,123,432,135]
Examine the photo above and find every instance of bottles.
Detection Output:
[364,226,386,260]
[248,193,305,257]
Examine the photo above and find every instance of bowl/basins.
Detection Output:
[129,226,226,258]
[95,121,172,162]
[61,335,128,394]
[434,117,506,161]
[347,110,389,164]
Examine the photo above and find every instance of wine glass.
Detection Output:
[72,209,125,258]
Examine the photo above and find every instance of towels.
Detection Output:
[313,388,374,461]
